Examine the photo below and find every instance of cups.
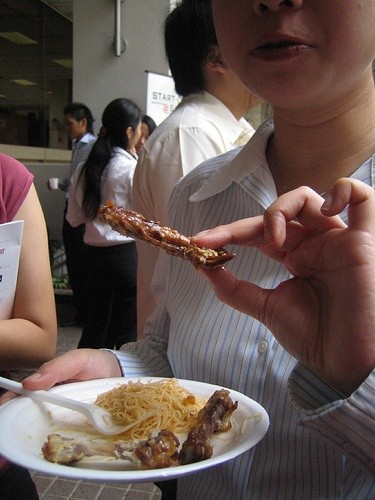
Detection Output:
[49,178,58,190]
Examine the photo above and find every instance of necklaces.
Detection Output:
[269,155,326,196]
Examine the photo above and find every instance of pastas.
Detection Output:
[94,378,207,440]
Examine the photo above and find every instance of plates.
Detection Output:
[0,376,269,482]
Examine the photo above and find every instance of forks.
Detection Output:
[0,376,162,436]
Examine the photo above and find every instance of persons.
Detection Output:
[66,98,143,349]
[46,103,98,324]
[131,0,268,500]
[0,152,58,500]
[0,0,375,500]
[136,115,157,155]
